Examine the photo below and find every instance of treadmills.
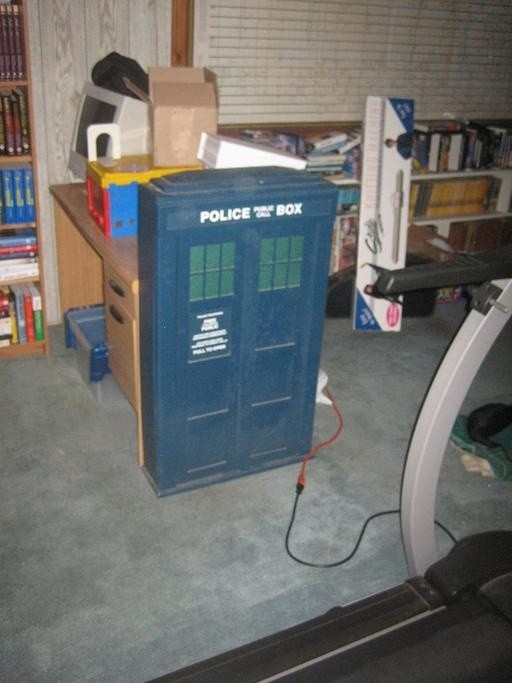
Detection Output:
[144,251,512,682]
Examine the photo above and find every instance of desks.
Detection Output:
[49,182,144,466]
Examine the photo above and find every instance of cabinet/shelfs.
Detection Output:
[0,1,49,361]
[103,260,135,409]
[217,119,512,277]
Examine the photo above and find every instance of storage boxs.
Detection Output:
[66,306,125,406]
[122,67,220,167]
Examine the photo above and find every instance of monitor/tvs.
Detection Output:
[68,83,148,183]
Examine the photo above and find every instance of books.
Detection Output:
[235,118,511,275]
[0,0,50,361]
